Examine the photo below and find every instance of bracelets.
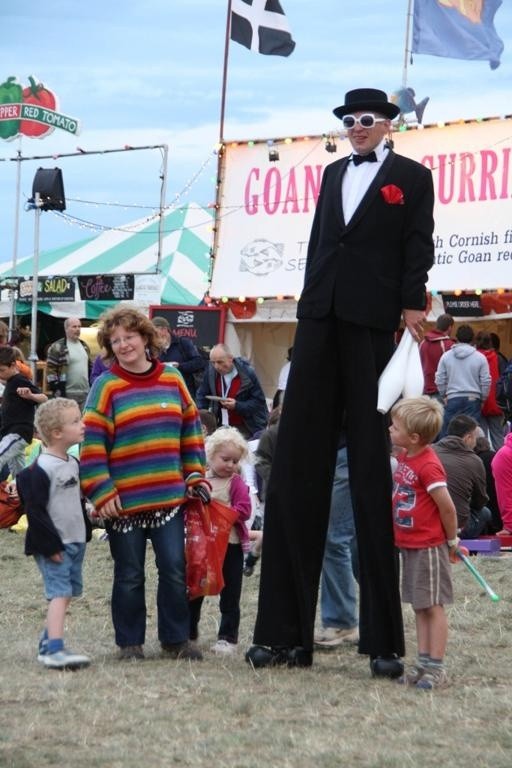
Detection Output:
[446,537,459,548]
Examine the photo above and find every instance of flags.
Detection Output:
[414,0,505,70]
[230,0,295,58]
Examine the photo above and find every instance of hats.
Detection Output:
[333,89,400,119]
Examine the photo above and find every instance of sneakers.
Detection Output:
[36,642,90,668]
[315,628,359,646]
[243,561,255,577]
[371,652,404,680]
[419,668,447,687]
[408,666,427,683]
[119,637,237,659]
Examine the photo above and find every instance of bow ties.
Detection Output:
[353,151,377,166]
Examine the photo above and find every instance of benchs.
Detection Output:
[478,535,512,548]
[458,538,501,556]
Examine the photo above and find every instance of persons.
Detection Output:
[196,343,270,440]
[77,309,212,664]
[184,426,251,655]
[1,314,113,480]
[270,346,293,409]
[15,397,91,669]
[245,424,364,648]
[247,87,434,675]
[148,316,205,400]
[198,405,283,577]
[419,315,512,538]
[386,397,462,687]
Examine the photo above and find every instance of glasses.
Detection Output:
[342,114,387,129]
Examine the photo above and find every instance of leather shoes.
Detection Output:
[245,645,314,669]
